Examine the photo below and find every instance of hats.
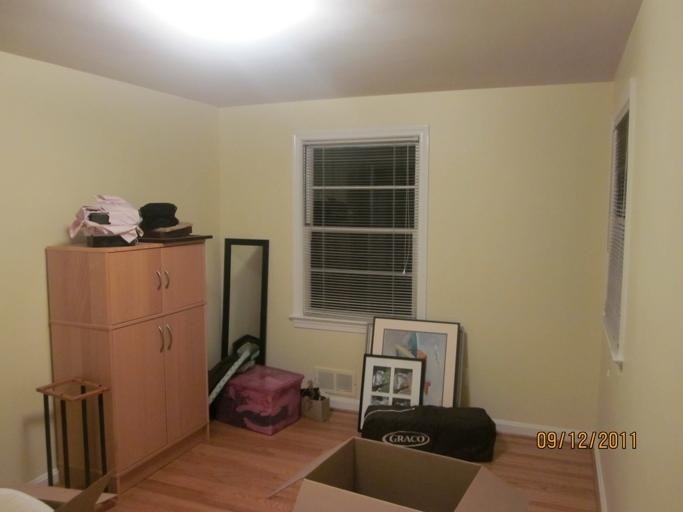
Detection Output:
[140,203,179,230]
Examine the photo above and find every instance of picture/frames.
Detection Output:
[356,354,424,431]
[370,316,460,408]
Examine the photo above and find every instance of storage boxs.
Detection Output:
[84,210,136,248]
[219,366,304,434]
[265,435,530,511]
[298,392,331,422]
[0,463,125,512]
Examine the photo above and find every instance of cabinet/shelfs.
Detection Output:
[44,234,214,497]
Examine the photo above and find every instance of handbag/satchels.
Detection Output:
[362,405,495,462]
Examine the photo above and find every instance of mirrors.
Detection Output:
[221,237,268,366]
